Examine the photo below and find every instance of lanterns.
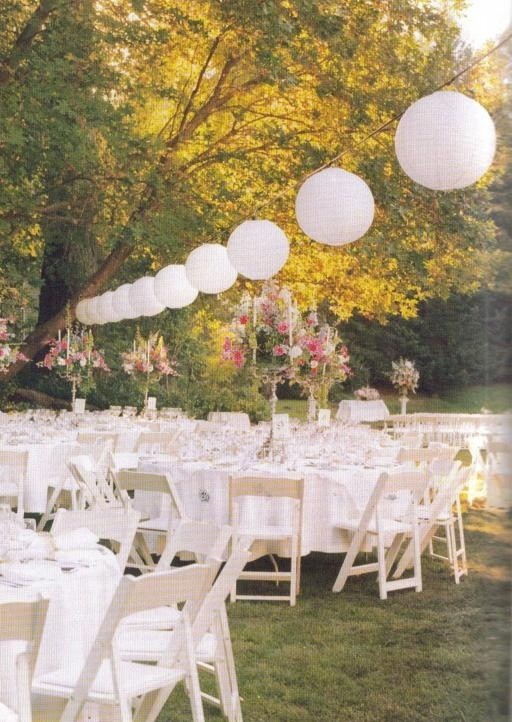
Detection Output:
[294,167,376,247]
[394,91,498,195]
[73,216,292,328]
